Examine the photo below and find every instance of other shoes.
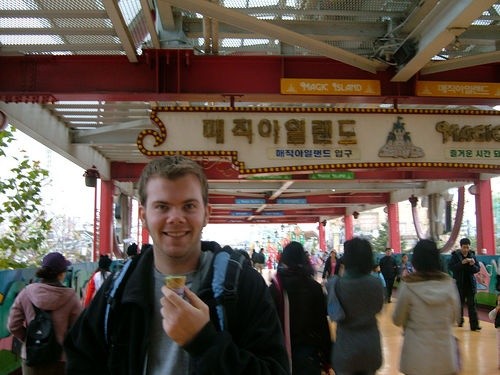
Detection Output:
[459,323,462,327]
[471,328,481,330]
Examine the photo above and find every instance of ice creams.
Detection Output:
[164,276,185,298]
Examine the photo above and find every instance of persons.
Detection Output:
[8,252,83,375]
[251,237,500,375]
[81,254,113,310]
[63,155,291,375]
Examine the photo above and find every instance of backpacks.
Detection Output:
[25,303,63,368]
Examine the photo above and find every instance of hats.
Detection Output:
[43,252,72,271]
